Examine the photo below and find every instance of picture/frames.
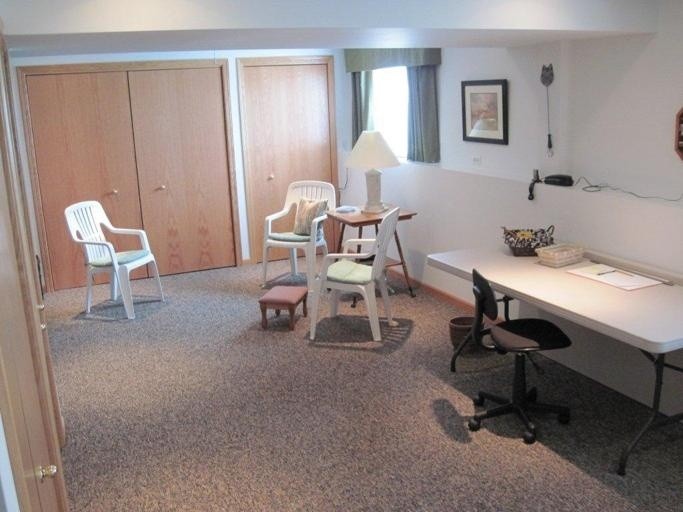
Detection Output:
[461,78,509,146]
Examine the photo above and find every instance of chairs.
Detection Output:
[64,200,165,320]
[310,206,401,341]
[260,180,336,294]
[468,267,572,445]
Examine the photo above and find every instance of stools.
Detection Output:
[258,285,308,331]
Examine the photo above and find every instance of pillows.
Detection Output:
[294,197,328,235]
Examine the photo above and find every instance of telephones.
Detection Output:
[543,171,573,187]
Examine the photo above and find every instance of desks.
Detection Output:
[426,240,683,477]
[326,207,418,308]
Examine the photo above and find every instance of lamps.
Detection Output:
[344,130,401,214]
